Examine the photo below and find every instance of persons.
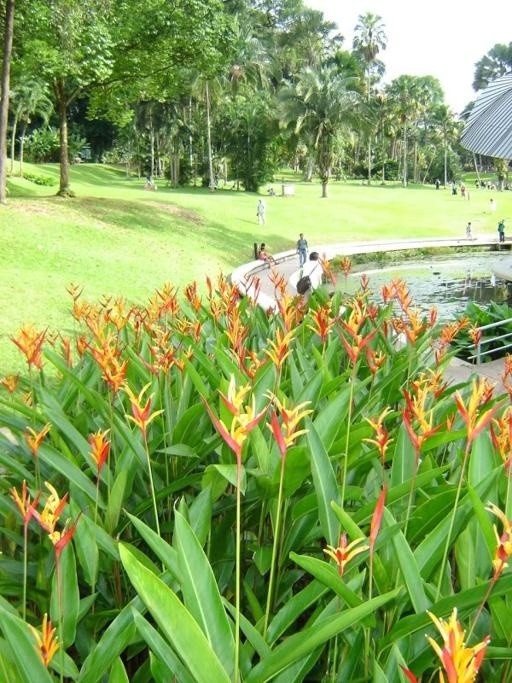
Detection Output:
[255,179,325,291]
[436,172,511,245]
[144,177,158,190]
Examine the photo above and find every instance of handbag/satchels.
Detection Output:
[297,276,311,294]
